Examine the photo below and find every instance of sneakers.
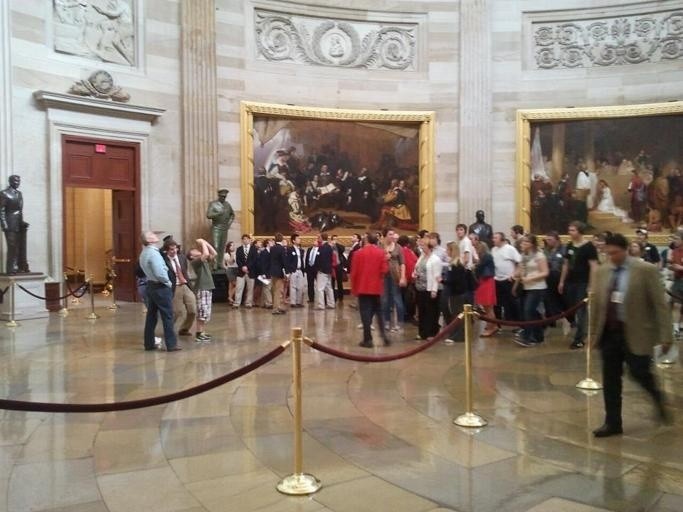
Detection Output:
[357,323,455,348]
[570,339,586,349]
[557,319,571,343]
[195,334,212,342]
[513,339,535,348]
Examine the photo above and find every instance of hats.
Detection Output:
[636,228,648,235]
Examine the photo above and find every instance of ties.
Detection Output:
[605,271,620,332]
[173,259,186,284]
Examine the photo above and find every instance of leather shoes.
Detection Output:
[179,329,192,336]
[168,345,183,352]
[513,328,525,333]
[593,419,624,438]
[479,328,500,339]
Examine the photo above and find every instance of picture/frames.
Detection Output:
[239,101,435,247]
[516,101,683,246]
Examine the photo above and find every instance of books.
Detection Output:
[652,342,679,364]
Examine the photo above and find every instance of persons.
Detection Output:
[470,221,600,351]
[136,229,217,353]
[265,146,418,233]
[0,174,31,275]
[529,149,682,235]
[468,210,492,251]
[206,188,235,271]
[252,233,347,315]
[347,227,481,348]
[223,241,237,305]
[232,234,257,308]
[591,230,681,315]
[590,428,669,512]
[586,233,676,437]
